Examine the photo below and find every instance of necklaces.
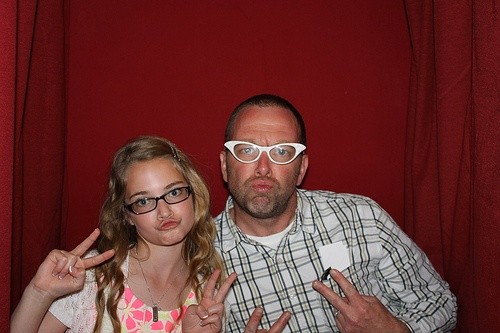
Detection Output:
[134,246,186,322]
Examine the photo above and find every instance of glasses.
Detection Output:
[124,186,190,215]
[225,140,307,165]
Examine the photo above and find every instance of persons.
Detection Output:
[198,93,456,333]
[10,135,237,333]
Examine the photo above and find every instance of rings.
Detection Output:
[59,250,67,255]
[334,312,338,318]
